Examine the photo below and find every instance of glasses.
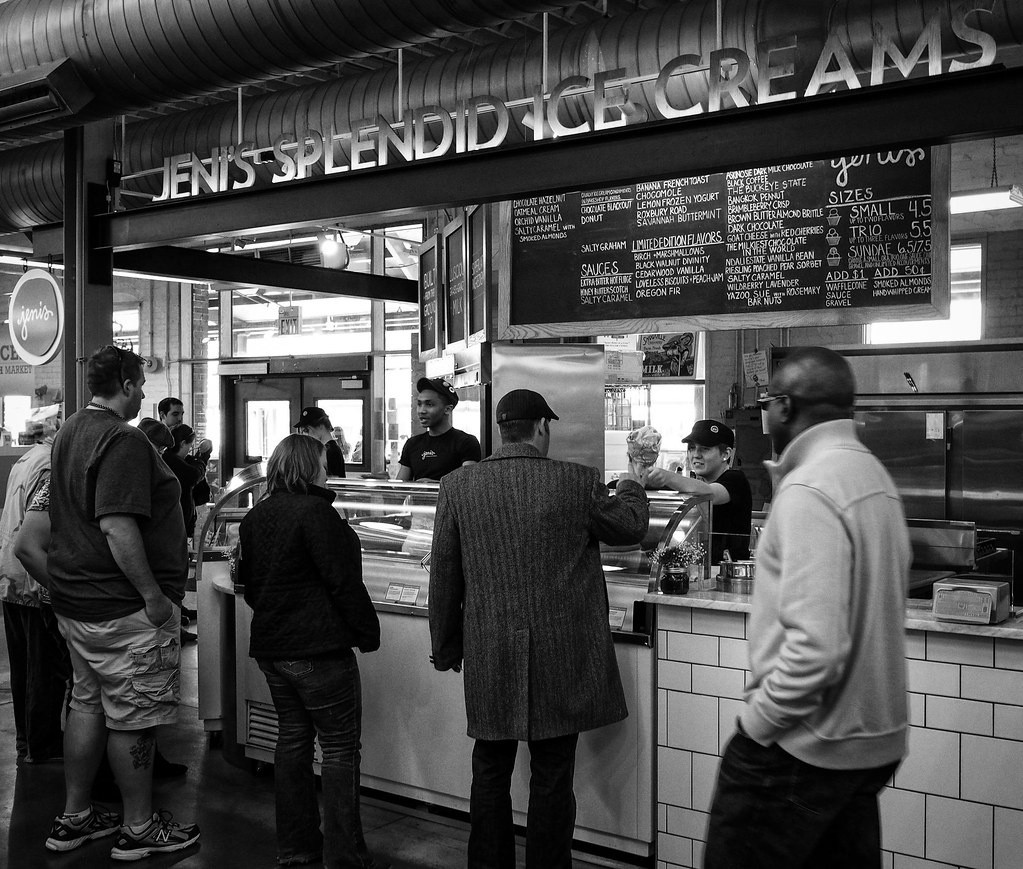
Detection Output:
[109,345,134,388]
[755,396,787,410]
[302,426,312,433]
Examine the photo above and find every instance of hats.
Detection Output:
[497,390,559,422]
[682,419,735,451]
[293,407,326,428]
[416,377,461,406]
[138,418,174,449]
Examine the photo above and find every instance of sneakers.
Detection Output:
[45,806,121,851]
[111,808,201,861]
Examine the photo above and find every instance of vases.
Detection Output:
[660,569,690,595]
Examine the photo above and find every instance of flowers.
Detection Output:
[647,535,709,568]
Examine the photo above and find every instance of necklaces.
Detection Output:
[87,401,126,422]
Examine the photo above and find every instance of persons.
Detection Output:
[157,397,185,429]
[47,346,203,859]
[331,426,363,462]
[650,419,751,566]
[429,388,651,869]
[137,417,176,457]
[160,424,213,646]
[0,432,74,869]
[292,406,350,522]
[701,346,913,869]
[233,436,380,869]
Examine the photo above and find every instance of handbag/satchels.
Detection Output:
[188,450,213,506]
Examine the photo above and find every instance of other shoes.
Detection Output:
[29,743,64,763]
[367,862,392,869]
[17,746,28,756]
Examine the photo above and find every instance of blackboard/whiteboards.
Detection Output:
[418,144,953,364]
